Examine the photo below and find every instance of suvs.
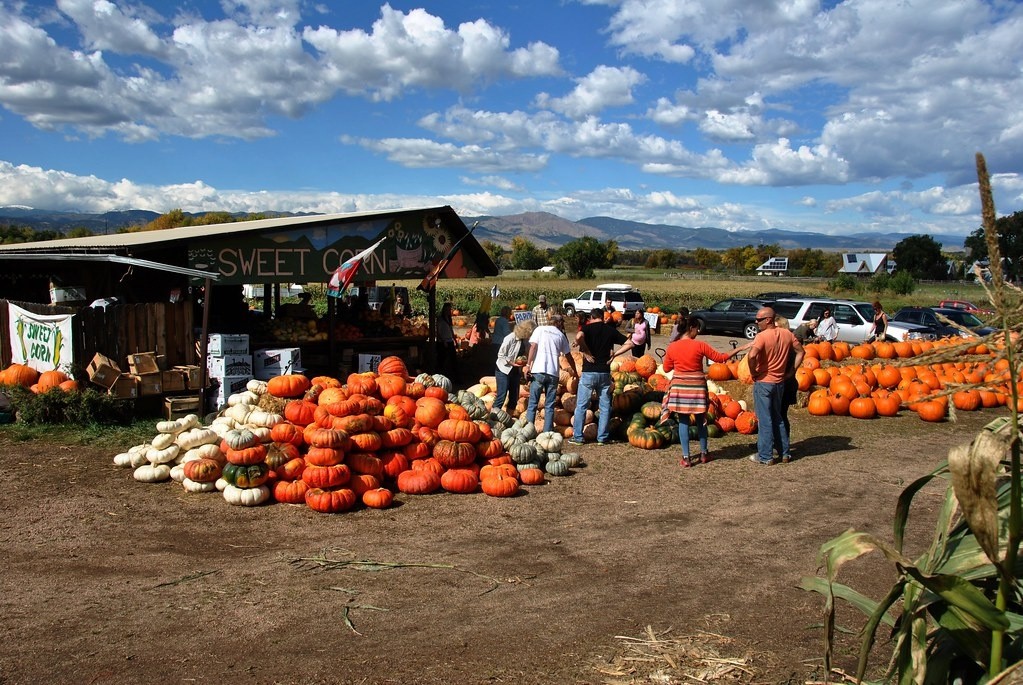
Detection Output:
[941,300,996,315]
[563,283,646,320]
[892,305,999,339]
[773,299,938,349]
[349,284,412,320]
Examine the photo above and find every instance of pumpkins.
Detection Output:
[356,309,431,336]
[0,359,78,394]
[259,318,363,341]
[450,303,526,355]
[467,351,699,449]
[795,330,1023,421]
[707,359,756,384]
[603,311,623,322]
[647,305,678,323]
[114,355,580,509]
[704,392,758,436]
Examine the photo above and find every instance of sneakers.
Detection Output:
[568,438,584,445]
[598,441,604,446]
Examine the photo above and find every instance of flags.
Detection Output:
[325,237,385,299]
[415,227,475,293]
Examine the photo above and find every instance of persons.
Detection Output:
[749,307,806,465]
[867,301,888,342]
[663,317,754,467]
[774,317,799,449]
[299,292,689,446]
[813,307,839,344]
[794,319,821,346]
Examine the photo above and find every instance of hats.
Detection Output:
[539,295,546,303]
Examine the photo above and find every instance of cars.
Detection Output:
[692,298,776,341]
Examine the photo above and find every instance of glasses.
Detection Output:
[756,317,772,322]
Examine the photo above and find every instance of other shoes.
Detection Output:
[749,454,774,466]
[780,455,789,463]
[680,457,691,469]
[701,453,714,463]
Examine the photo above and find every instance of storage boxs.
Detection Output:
[83,334,310,422]
[49,276,86,304]
[337,348,381,383]
